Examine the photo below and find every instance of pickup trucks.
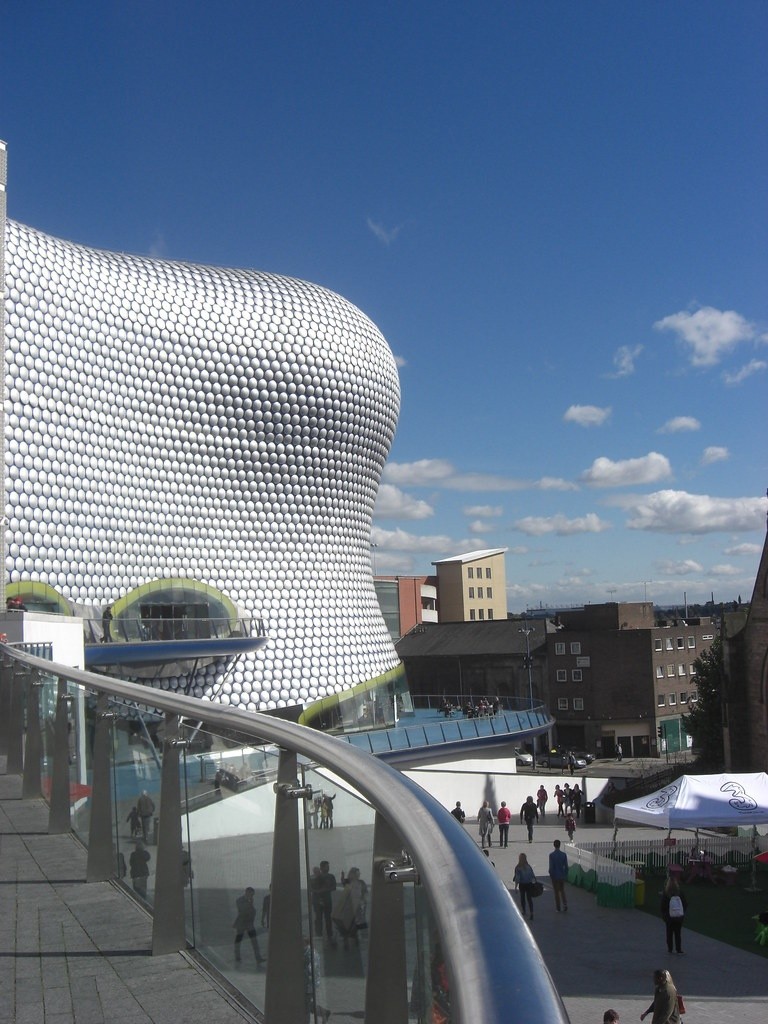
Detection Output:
[383,854,415,883]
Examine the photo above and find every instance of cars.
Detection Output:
[568,747,595,763]
[541,750,588,768]
[515,748,534,765]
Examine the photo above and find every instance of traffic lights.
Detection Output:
[658,726,663,738]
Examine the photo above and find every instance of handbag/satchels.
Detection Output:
[531,877,543,897]
[677,995,685,1015]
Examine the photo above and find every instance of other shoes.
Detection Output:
[677,951,684,953]
[668,950,672,953]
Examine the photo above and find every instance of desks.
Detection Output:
[685,859,718,885]
[624,861,646,885]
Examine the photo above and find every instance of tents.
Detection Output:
[611,772,768,863]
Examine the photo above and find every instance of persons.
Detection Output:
[100,606,113,642]
[126,789,155,838]
[483,850,495,867]
[549,839,569,912]
[513,853,534,920]
[661,877,687,953]
[640,969,682,1024]
[568,755,575,775]
[306,784,336,829]
[6,596,28,611]
[440,696,500,718]
[309,860,367,949]
[215,762,250,796]
[614,743,623,761]
[520,782,584,843]
[233,885,272,964]
[603,1009,619,1024]
[130,841,151,898]
[451,801,511,848]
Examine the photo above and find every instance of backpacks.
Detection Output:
[669,894,684,917]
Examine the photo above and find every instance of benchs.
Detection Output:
[668,863,684,881]
[721,870,738,885]
[635,879,645,905]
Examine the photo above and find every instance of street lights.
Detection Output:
[518,615,539,770]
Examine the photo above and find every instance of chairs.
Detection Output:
[468,704,503,720]
